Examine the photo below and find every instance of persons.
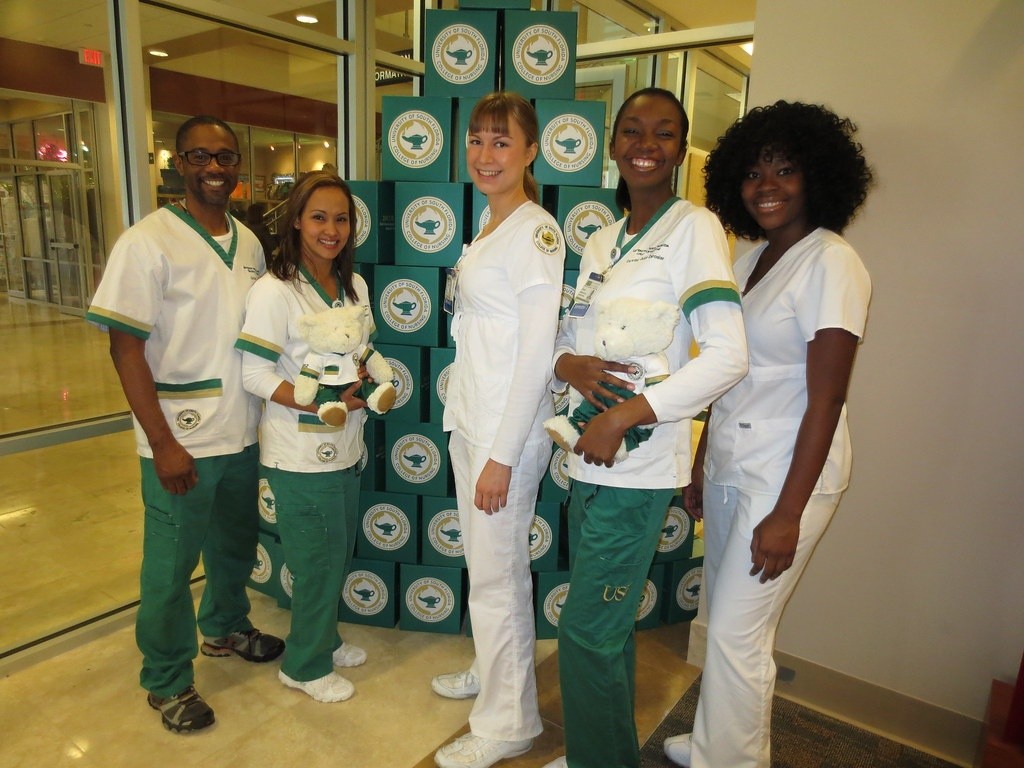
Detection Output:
[233,170,378,703]
[431,92,567,768]
[85,115,285,732]
[552,87,747,768]
[664,100,873,768]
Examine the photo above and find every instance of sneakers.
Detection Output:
[201,628,285,663]
[434,731,534,768]
[278,669,355,703]
[664,732,693,767]
[333,642,367,667]
[148,686,216,733]
[431,670,481,700]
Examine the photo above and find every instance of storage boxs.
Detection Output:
[392,181,471,267]
[541,442,573,504]
[550,380,571,419]
[543,184,623,271]
[1002,653,1024,737]
[661,535,712,626]
[422,495,468,568]
[257,463,280,535]
[633,564,666,631]
[498,8,578,101]
[348,262,375,286]
[398,564,468,636]
[532,560,575,641]
[468,182,545,243]
[381,95,458,182]
[343,178,396,266]
[385,422,456,497]
[457,97,486,184]
[273,540,295,609]
[359,419,386,491]
[649,495,696,565]
[558,269,582,329]
[338,557,400,630]
[445,310,458,349]
[527,491,563,572]
[424,7,501,96]
[428,346,457,425]
[975,677,1024,768]
[456,0,533,12]
[245,527,283,598]
[372,266,450,347]
[533,98,607,188]
[362,343,430,425]
[357,490,423,564]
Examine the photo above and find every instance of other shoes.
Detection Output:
[542,756,568,768]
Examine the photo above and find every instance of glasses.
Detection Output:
[179,151,241,167]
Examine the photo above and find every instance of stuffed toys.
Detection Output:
[545,298,678,464]
[294,305,396,426]
